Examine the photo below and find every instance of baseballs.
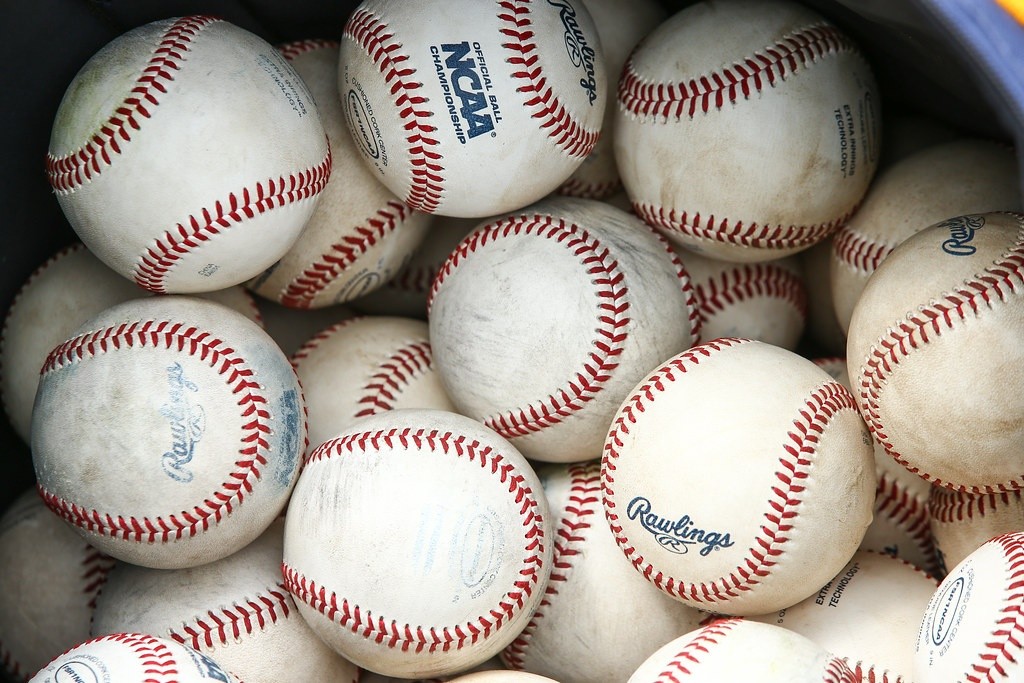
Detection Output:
[0,0,1022,681]
